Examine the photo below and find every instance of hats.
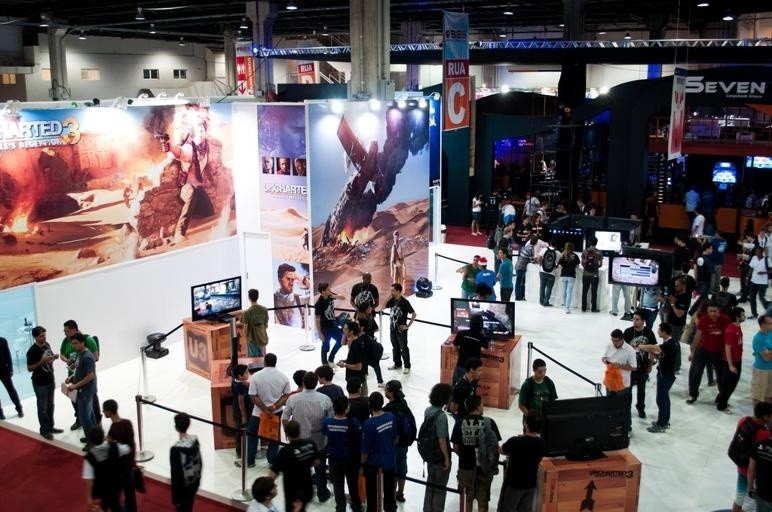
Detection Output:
[347,376,364,389]
[386,381,405,398]
[477,257,488,266]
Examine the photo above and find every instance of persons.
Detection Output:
[732,401,771,511]
[455,186,604,313]
[602,183,771,433]
[229,272,558,511]
[1,319,203,511]
[262,155,306,173]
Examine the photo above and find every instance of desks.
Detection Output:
[525,251,612,311]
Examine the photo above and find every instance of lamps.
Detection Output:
[40,7,249,47]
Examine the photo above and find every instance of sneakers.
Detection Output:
[647,421,671,433]
[687,397,697,403]
[235,460,255,467]
[378,382,384,388]
[404,368,409,374]
[714,400,733,414]
[621,313,632,320]
[320,489,330,501]
[328,360,336,367]
[395,491,405,502]
[388,364,401,370]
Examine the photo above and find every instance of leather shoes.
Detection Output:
[80,437,88,442]
[637,406,645,417]
[40,431,53,439]
[18,409,24,418]
[83,443,92,451]
[71,422,81,430]
[50,428,63,433]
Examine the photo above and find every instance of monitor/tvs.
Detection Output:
[541,394,631,461]
[711,161,738,185]
[608,248,673,287]
[191,276,242,322]
[450,298,515,339]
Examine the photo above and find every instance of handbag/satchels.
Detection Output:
[680,324,694,343]
[478,430,499,475]
[416,410,451,464]
[134,468,145,493]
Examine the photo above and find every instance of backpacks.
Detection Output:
[542,247,556,273]
[582,249,598,272]
[388,403,416,446]
[728,416,767,467]
[366,336,383,364]
[715,292,732,313]
[486,229,500,249]
